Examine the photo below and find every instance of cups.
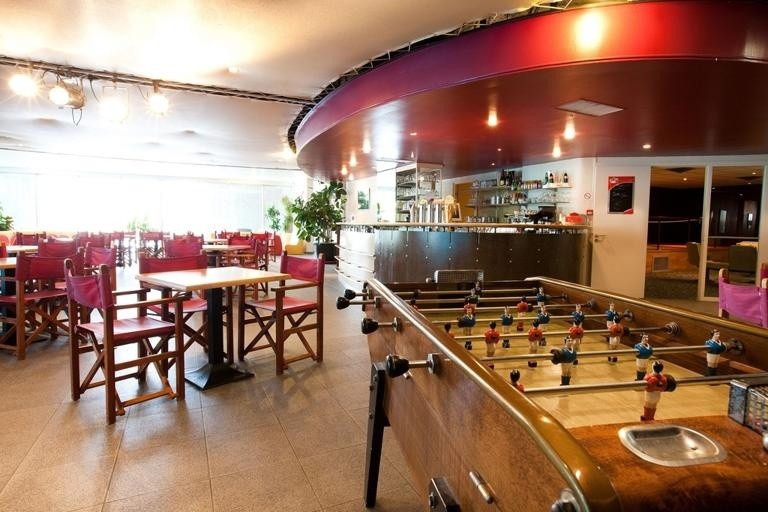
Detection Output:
[490,195,501,205]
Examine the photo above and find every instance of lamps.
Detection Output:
[9,61,168,124]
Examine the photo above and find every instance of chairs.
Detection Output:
[687,241,730,288]
[728,241,758,272]
[717,265,767,329]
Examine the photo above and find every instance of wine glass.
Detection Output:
[537,192,570,202]
[473,216,495,233]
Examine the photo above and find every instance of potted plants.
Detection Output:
[289,178,347,265]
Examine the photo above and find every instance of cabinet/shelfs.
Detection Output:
[396,162,442,224]
[466,185,572,223]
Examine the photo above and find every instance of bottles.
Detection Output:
[586,210,593,226]
[499,168,568,189]
